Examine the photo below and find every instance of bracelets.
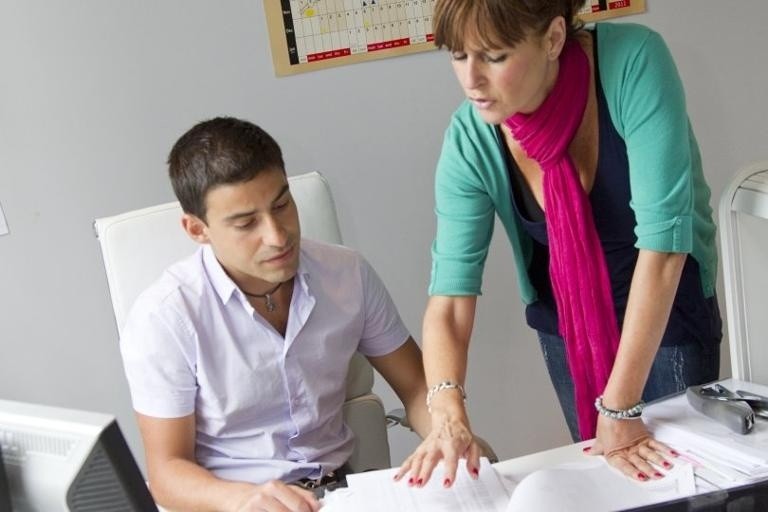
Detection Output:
[424,380,466,416]
[601,404,643,420]
[593,394,645,422]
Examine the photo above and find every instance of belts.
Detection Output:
[295,464,351,491]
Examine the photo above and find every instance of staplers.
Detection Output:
[686,384,768,434]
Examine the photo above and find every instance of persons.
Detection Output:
[118,109,502,512]
[391,0,725,490]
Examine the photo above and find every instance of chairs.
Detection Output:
[91,169,499,497]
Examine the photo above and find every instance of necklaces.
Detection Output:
[237,280,282,313]
